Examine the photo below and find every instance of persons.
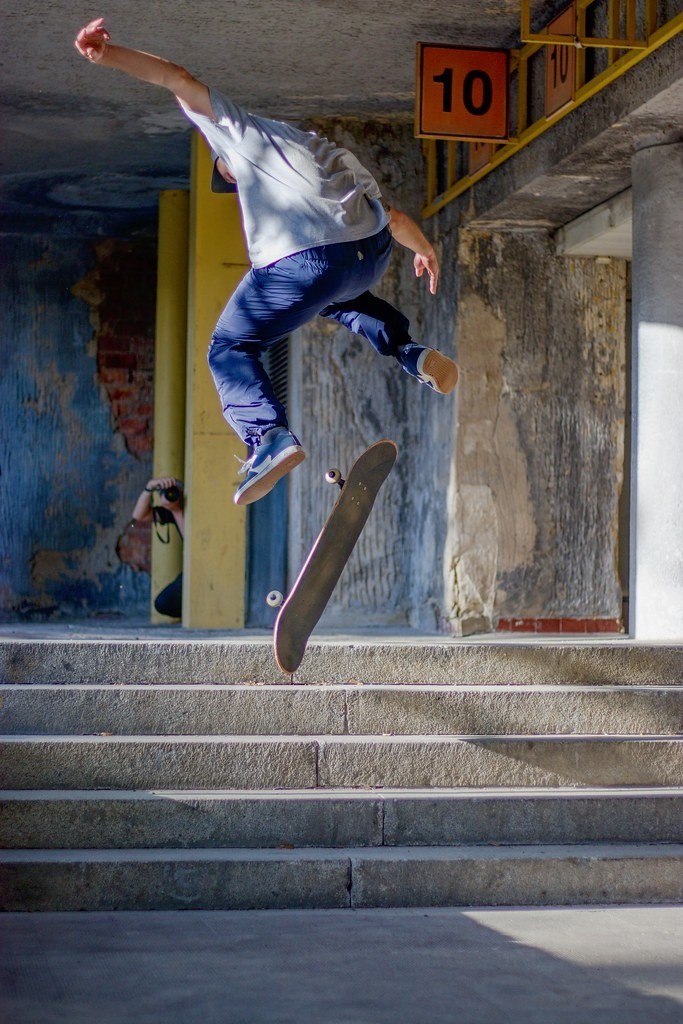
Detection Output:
[133,474,184,618]
[75,17,459,505]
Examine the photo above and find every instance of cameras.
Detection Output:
[159,485,180,502]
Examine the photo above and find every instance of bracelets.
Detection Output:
[145,486,154,493]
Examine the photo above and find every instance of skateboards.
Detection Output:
[266,441,398,673]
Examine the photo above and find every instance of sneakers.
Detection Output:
[232,431,307,505]
[401,339,458,393]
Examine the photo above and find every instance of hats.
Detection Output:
[209,147,238,194]
